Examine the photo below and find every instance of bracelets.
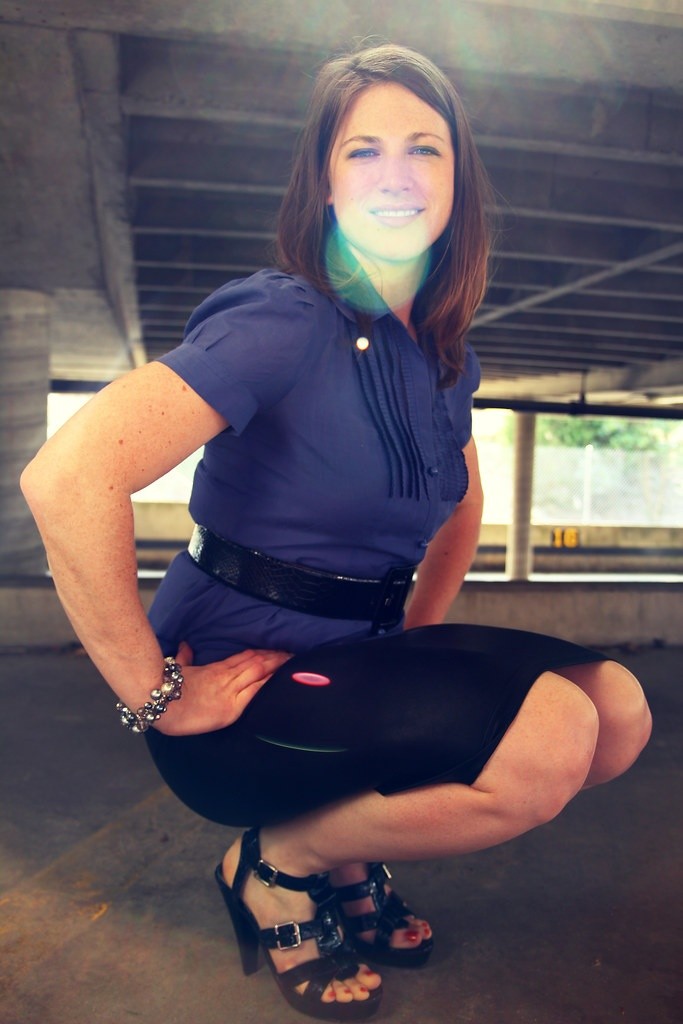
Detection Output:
[113,657,185,735]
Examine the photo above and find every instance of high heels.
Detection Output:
[215,826,384,1020]
[322,857,434,968]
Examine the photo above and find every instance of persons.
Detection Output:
[14,40,655,1023]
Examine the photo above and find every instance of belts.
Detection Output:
[186,525,414,632]
